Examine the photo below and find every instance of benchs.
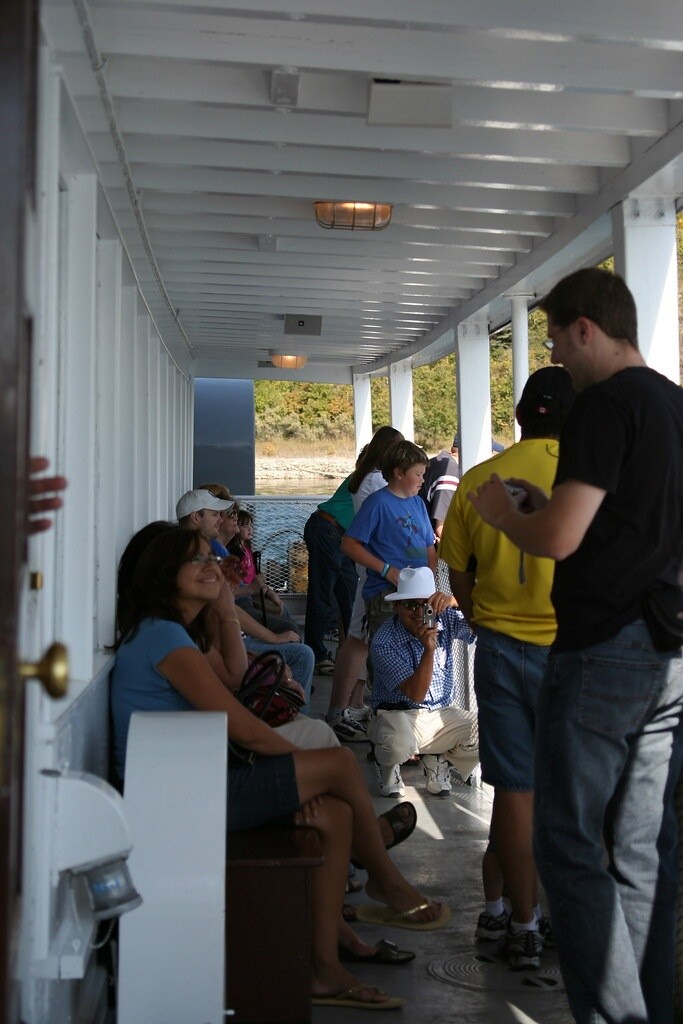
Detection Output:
[225,822,326,1024]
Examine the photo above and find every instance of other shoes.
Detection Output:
[313,651,337,676]
[534,915,558,945]
[323,629,340,642]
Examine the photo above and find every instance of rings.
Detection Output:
[440,599,445,603]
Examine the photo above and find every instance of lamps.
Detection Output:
[312,200,392,230]
[271,354,307,369]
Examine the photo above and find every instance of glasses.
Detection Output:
[399,599,428,611]
[221,509,238,517]
[542,321,578,350]
[188,555,223,565]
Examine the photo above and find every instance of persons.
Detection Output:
[107,521,452,1009]
[417,432,504,550]
[340,440,437,765]
[28,456,67,535]
[366,566,480,799]
[437,367,581,972]
[325,426,423,743]
[467,268,683,1024]
[304,444,369,676]
[176,484,316,716]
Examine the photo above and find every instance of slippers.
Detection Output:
[351,802,417,870]
[356,895,453,931]
[310,984,406,1008]
[337,938,415,965]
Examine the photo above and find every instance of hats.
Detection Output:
[453,430,505,453]
[176,490,235,522]
[517,366,574,418]
[384,567,436,600]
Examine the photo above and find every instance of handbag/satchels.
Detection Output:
[229,648,306,766]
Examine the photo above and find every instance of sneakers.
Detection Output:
[347,705,373,721]
[475,906,512,940]
[375,761,405,798]
[419,755,452,795]
[507,924,545,967]
[323,708,370,742]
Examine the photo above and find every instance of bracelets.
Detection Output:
[265,590,268,599]
[379,563,389,578]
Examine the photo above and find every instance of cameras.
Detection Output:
[422,603,437,629]
[509,485,531,508]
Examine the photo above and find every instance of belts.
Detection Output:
[316,509,345,536]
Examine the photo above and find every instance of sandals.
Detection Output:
[345,879,363,894]
[341,903,357,922]
